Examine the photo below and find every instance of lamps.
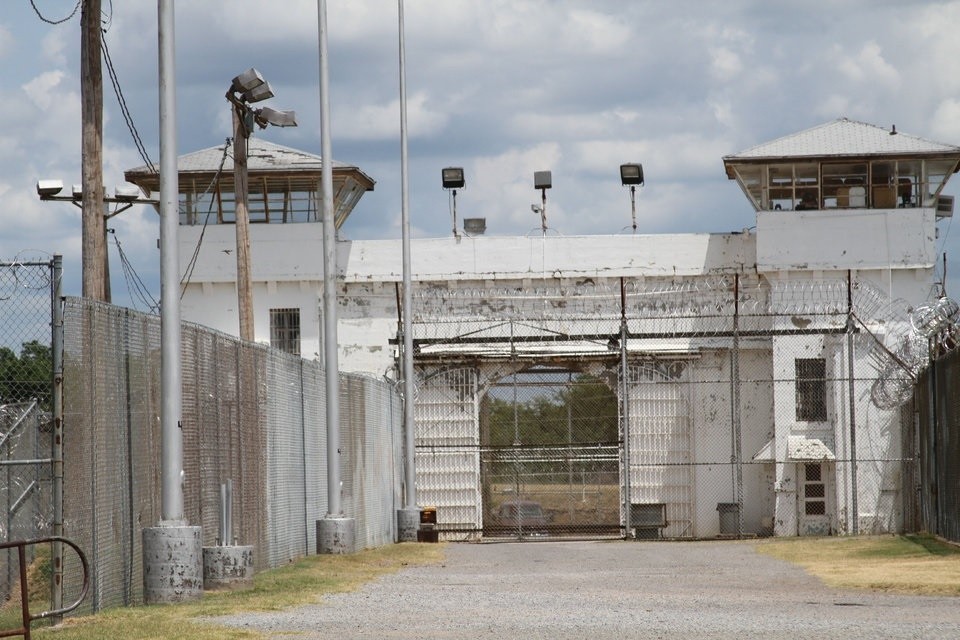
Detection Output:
[620,162,643,233]
[441,166,465,239]
[532,170,552,236]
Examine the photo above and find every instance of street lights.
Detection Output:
[33,179,162,306]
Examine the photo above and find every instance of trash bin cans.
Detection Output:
[716,503,740,533]
